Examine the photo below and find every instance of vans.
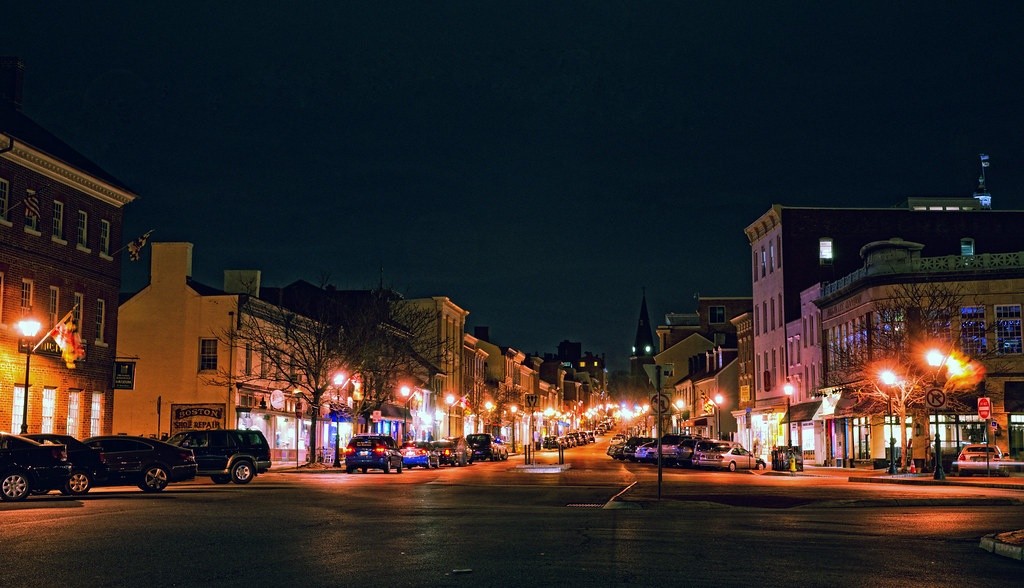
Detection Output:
[692,440,744,467]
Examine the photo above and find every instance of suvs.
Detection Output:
[345,433,404,475]
[167,429,273,484]
[655,433,703,467]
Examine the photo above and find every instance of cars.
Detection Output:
[754,457,766,470]
[957,443,1010,477]
[0,431,74,501]
[81,435,199,494]
[402,431,508,470]
[542,413,658,463]
[676,439,701,468]
[699,445,756,472]
[17,434,112,499]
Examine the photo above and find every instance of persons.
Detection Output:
[427,431,434,443]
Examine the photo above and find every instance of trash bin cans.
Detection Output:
[771,446,798,471]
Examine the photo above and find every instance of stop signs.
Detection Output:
[977,397,991,420]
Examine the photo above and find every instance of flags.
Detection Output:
[127,230,151,262]
[414,385,425,400]
[50,310,86,363]
[349,370,365,392]
[459,393,473,414]
[23,193,41,222]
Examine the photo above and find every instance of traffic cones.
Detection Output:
[910,459,918,474]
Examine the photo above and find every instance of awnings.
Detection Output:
[780,390,901,424]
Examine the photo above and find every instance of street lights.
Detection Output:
[784,382,794,459]
[400,385,411,448]
[925,348,945,479]
[676,398,685,436]
[15,311,42,435]
[511,405,518,453]
[880,371,900,476]
[715,394,723,441]
[446,392,454,438]
[332,371,346,469]
